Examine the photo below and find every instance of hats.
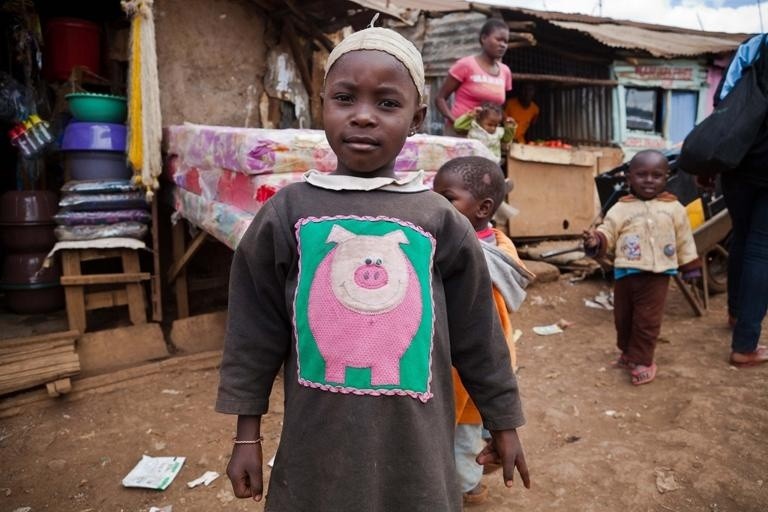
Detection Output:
[324,27,425,107]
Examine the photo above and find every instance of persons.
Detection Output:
[434,18,513,136]
[452,103,517,168]
[433,157,538,505]
[714,35,768,366]
[216,27,530,511]
[581,150,700,386]
[502,79,540,144]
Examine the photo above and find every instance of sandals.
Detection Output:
[730,345,768,368]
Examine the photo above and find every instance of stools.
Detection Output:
[55,237,148,333]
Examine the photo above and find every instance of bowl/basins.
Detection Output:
[64,92,127,122]
[61,123,126,151]
[63,151,128,184]
[0,190,67,313]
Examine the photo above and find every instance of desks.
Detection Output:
[503,144,597,238]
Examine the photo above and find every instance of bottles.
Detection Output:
[9,114,54,160]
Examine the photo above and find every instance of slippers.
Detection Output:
[619,354,634,367]
[631,362,657,385]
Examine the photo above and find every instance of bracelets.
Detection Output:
[231,437,265,444]
